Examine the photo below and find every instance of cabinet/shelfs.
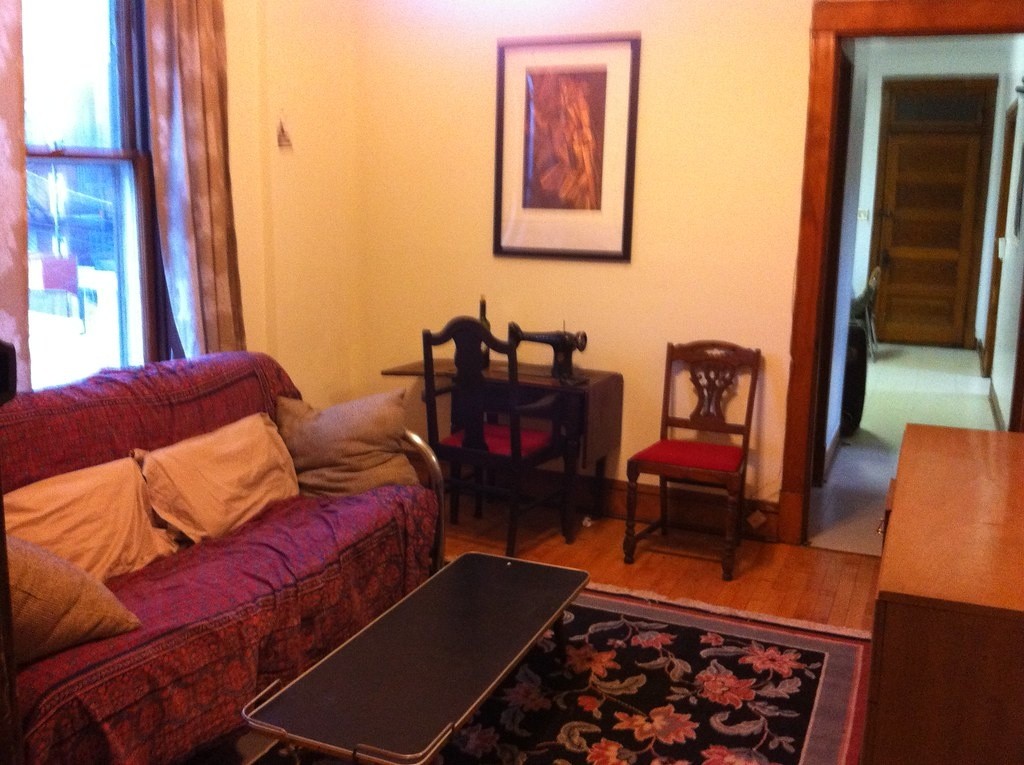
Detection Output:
[859,421,1024,764]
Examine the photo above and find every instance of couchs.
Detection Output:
[0,349,446,764]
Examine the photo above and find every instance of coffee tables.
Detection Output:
[240,548,590,763]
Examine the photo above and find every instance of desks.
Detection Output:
[380,357,624,542]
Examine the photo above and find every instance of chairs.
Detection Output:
[622,339,761,580]
[421,314,589,558]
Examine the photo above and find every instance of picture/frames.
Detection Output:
[493,32,645,264]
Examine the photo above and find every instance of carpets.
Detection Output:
[246,579,874,765]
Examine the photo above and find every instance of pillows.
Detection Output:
[0,457,180,584]
[6,532,142,665]
[141,411,300,544]
[272,388,419,499]
